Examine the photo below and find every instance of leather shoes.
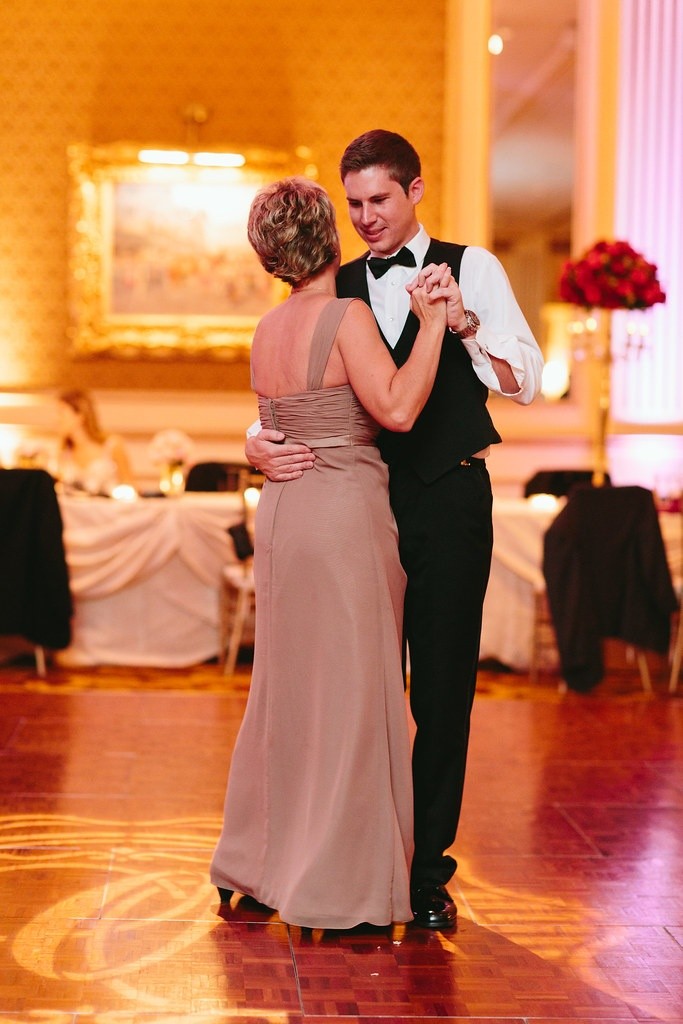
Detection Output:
[411,884,457,927]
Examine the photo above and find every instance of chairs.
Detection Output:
[0,468,78,677]
[524,470,612,498]
[186,462,265,493]
[221,485,255,678]
[528,484,683,701]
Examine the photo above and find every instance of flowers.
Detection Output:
[557,238,667,314]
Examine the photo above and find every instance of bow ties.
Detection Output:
[367,246,417,279]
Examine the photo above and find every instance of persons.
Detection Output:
[209,175,448,939]
[49,386,138,494]
[244,129,543,928]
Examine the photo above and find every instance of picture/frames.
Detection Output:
[60,139,317,363]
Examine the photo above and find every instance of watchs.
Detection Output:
[448,310,479,339]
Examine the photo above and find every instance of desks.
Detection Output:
[474,496,683,681]
[0,490,257,671]
